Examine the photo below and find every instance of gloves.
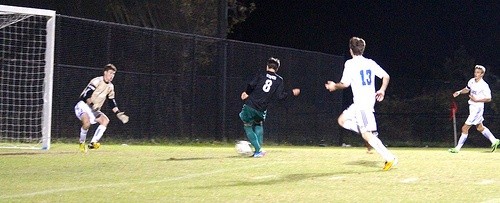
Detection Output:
[91,107,103,121]
[114,110,130,124]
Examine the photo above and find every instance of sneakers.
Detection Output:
[79,142,86,152]
[88,142,100,149]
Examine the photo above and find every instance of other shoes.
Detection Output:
[251,152,263,157]
[448,147,458,153]
[491,140,500,153]
[382,159,398,171]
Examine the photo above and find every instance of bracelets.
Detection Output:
[115,111,119,115]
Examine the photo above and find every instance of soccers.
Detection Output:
[235,141,251,155]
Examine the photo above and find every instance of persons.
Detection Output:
[325,37,398,171]
[448,65,500,153]
[239,57,300,157]
[75,64,129,153]
